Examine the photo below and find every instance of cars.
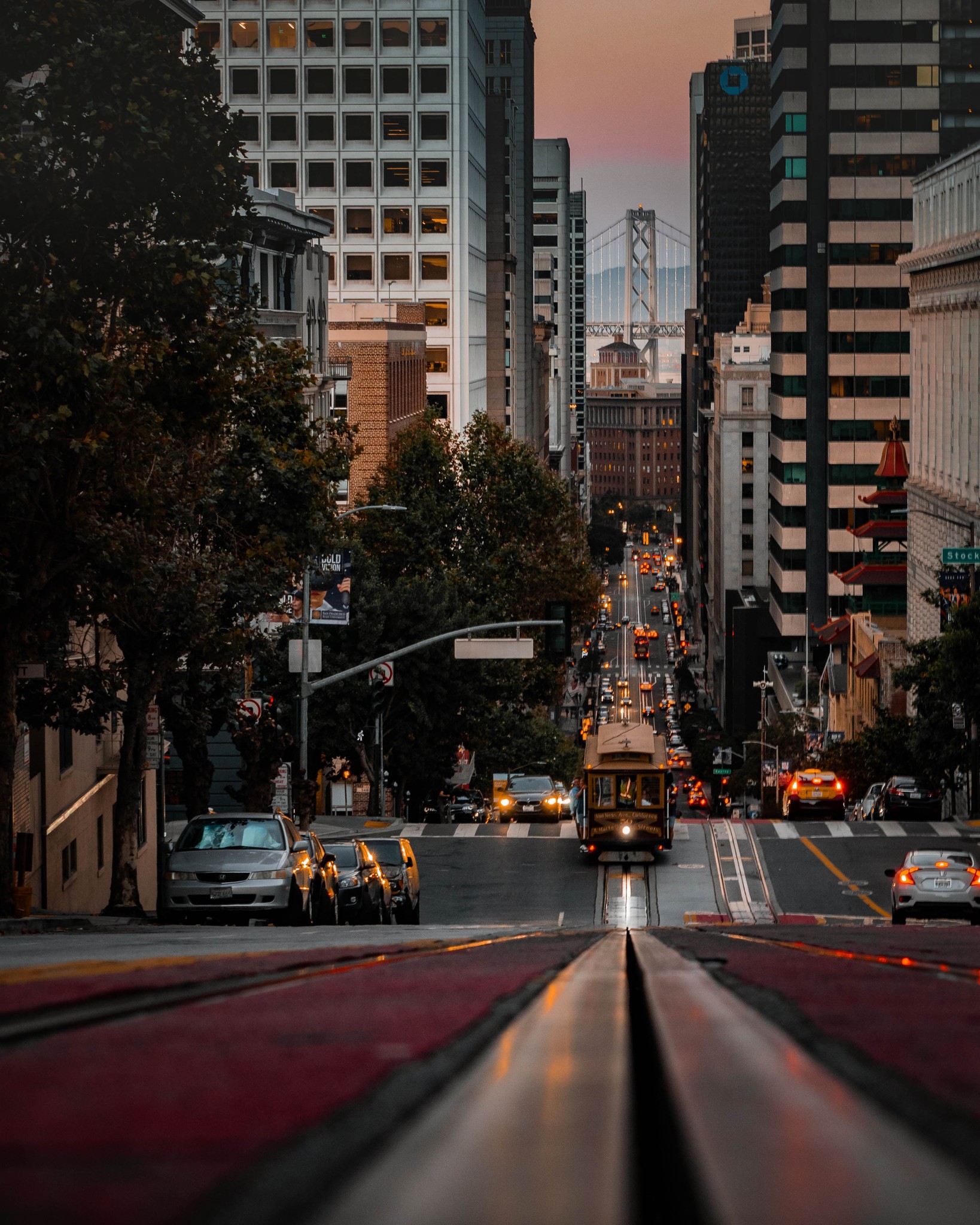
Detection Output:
[850,776,942,823]
[556,786,571,820]
[592,542,707,810]
[487,803,500,824]
[420,787,492,823]
[296,831,422,926]
[779,769,846,821]
[497,775,564,824]
[884,850,980,926]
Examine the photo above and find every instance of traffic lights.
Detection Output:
[355,726,376,744]
[370,679,386,714]
[724,797,729,804]
[722,778,727,785]
[259,694,274,711]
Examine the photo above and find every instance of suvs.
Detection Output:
[158,805,313,927]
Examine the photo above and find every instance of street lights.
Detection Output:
[779,710,823,765]
[718,750,746,819]
[507,762,547,782]
[298,503,408,832]
[741,740,780,819]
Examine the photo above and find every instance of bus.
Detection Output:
[574,717,673,865]
